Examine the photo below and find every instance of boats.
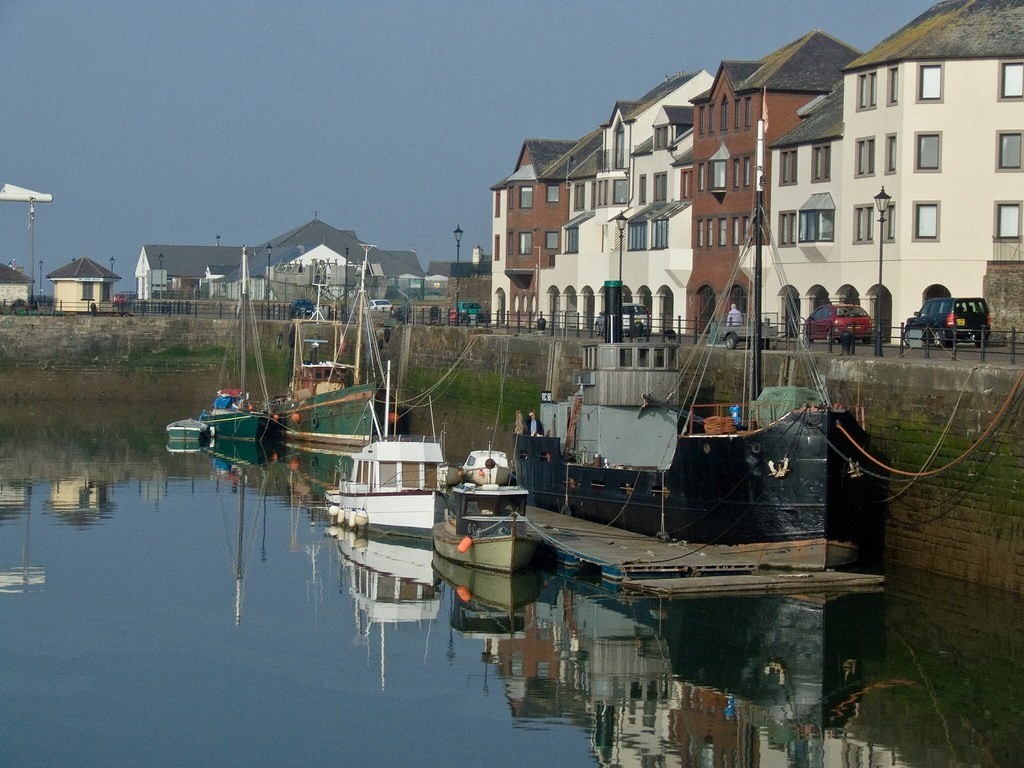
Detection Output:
[325,442,455,539]
[329,520,442,692]
[163,246,379,448]
[430,550,541,694]
[547,574,891,768]
[165,439,354,625]
[433,485,540,572]
[511,119,873,573]
[462,450,510,483]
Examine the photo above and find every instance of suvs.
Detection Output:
[595,303,652,338]
[902,296,991,348]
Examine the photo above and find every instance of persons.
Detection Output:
[726,303,743,326]
[524,411,544,437]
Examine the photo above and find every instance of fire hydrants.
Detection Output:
[451,308,457,326]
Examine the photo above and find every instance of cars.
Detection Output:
[288,299,315,315]
[112,293,128,303]
[803,304,874,345]
[447,300,483,323]
[363,300,394,317]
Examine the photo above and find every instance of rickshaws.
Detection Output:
[717,326,779,351]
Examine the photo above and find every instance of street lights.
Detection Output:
[158,251,164,315]
[615,211,629,344]
[453,225,464,327]
[38,259,43,307]
[109,256,116,273]
[873,184,894,357]
[266,241,272,320]
